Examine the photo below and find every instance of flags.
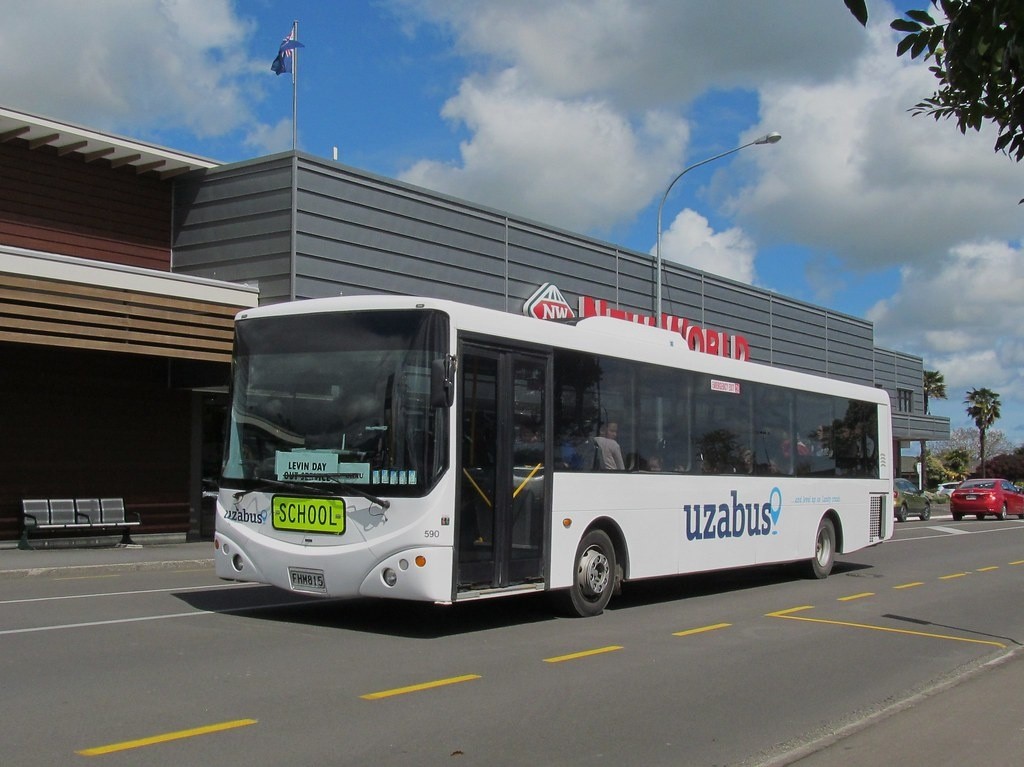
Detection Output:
[271,26,305,75]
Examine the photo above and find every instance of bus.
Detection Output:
[216,294,894,616]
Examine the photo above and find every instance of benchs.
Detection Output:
[18,498,141,551]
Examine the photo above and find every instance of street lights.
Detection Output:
[656,131,783,329]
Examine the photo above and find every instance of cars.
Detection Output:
[893,477,931,523]
[937,482,962,498]
[951,479,1024,520]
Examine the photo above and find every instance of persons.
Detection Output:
[594,423,625,470]
[781,422,875,460]
